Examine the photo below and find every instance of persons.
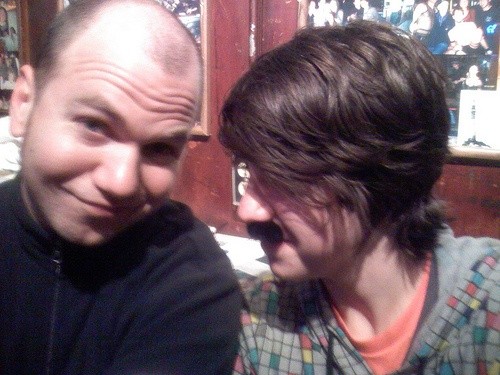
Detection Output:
[219,16,500,374]
[300,0,495,91]
[1,1,245,375]
[1,16,21,79]
